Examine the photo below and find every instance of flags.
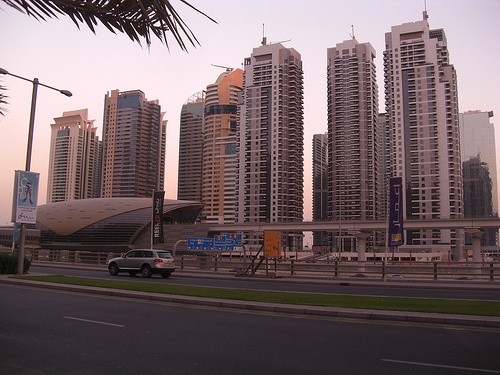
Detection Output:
[391,178,405,246]
[153,191,165,245]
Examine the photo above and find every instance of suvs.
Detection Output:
[108,249,176,279]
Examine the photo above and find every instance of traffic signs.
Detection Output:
[186,232,244,251]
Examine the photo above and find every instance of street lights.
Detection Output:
[0,69,72,278]
